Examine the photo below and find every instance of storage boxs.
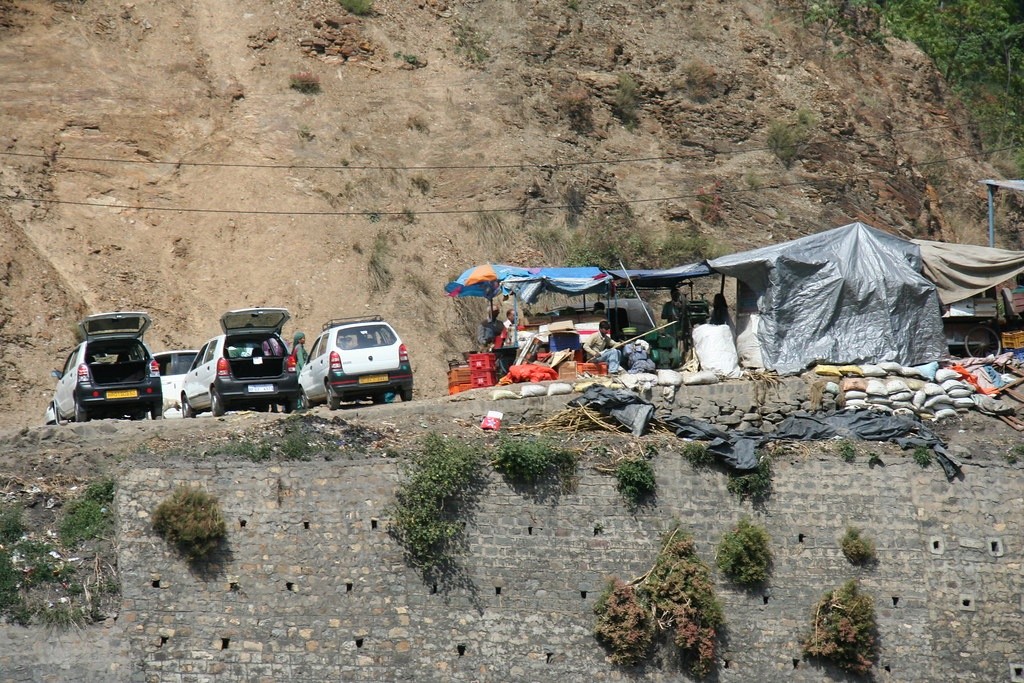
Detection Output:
[520,313,607,331]
[447,368,469,396]
[468,353,497,388]
[555,360,576,378]
[549,334,579,352]
[1000,329,1024,364]
[936,295,997,316]
[577,362,608,377]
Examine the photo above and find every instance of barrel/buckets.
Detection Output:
[548,333,580,353]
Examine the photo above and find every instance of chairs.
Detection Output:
[116,351,129,363]
[251,347,265,356]
[355,336,371,348]
[87,357,96,363]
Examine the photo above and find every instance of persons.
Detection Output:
[661,289,689,337]
[263,338,286,412]
[292,333,308,408]
[340,337,352,348]
[502,309,518,328]
[584,322,626,377]
[485,306,506,332]
[1013,273,1024,320]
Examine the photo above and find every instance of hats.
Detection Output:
[599,321,611,329]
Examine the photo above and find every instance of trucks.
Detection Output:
[551,298,656,334]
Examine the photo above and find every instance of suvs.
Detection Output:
[297,311,415,406]
[40,307,200,428]
[179,307,301,417]
